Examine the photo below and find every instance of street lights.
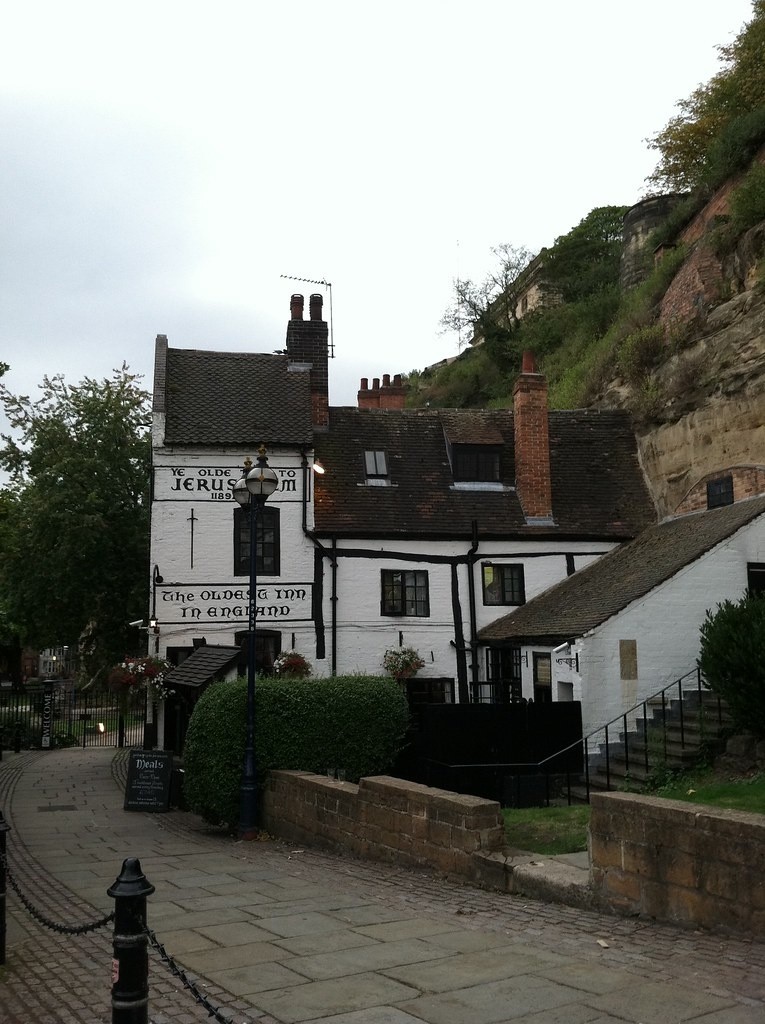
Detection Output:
[234,446,282,839]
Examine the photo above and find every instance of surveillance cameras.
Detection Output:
[552,641,569,654]
[129,619,143,627]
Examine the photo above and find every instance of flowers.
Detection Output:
[381,645,424,677]
[271,649,311,680]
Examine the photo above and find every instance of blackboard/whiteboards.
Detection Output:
[123,748,172,813]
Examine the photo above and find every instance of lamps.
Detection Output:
[312,448,326,475]
[149,614,158,628]
